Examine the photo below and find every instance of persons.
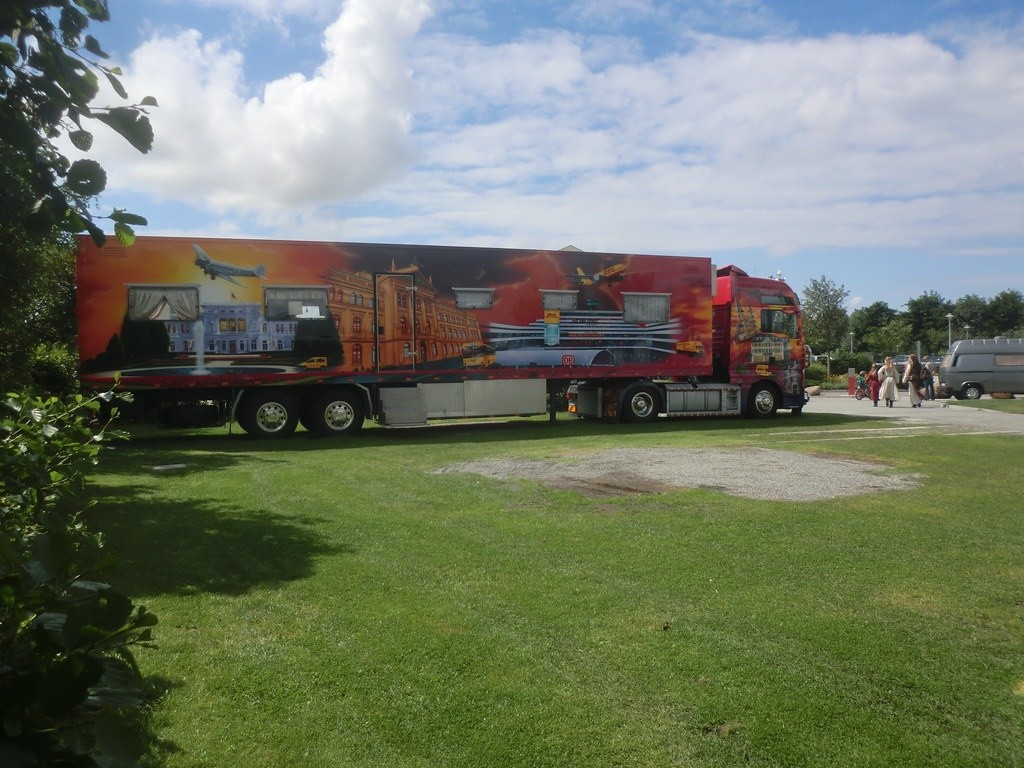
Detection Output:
[857,354,935,407]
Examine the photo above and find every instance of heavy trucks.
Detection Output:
[74,229,814,439]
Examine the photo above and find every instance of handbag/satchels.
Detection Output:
[920,365,927,380]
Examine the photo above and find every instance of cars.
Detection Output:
[807,354,834,363]
[872,354,944,386]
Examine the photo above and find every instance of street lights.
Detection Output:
[945,312,954,350]
[962,324,971,340]
[849,331,855,354]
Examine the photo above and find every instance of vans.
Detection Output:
[938,335,1024,399]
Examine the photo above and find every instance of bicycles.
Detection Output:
[855,386,870,400]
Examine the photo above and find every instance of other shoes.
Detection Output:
[918,404,921,406]
[886,403,888,406]
[873,404,877,407]
[911,404,916,408]
[889,406,892,408]
